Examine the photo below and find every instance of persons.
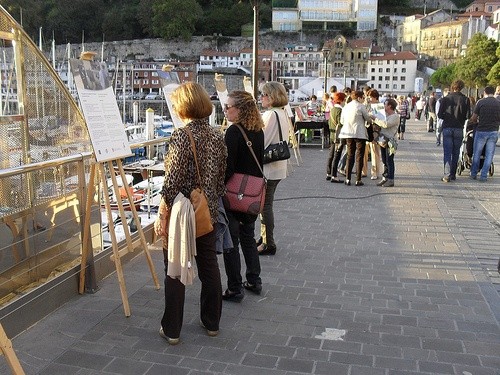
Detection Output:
[255,81,289,255]
[438,80,470,182]
[223,90,265,302]
[469,86,500,180]
[366,89,384,180]
[153,80,229,344]
[306,95,319,114]
[338,90,372,186]
[329,85,500,177]
[370,99,400,187]
[325,92,347,183]
[322,94,332,120]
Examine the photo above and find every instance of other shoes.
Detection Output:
[199,318,219,335]
[436,142,440,146]
[337,169,346,176]
[160,326,179,344]
[355,181,364,186]
[441,177,455,182]
[256,238,276,255]
[222,288,243,303]
[242,281,262,295]
[345,180,351,185]
[472,175,487,181]
[326,176,344,183]
[377,179,394,187]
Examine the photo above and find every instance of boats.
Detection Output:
[34,115,175,249]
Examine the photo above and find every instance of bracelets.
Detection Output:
[158,213,165,219]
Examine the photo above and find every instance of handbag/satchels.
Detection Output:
[225,172,266,216]
[262,111,290,165]
[161,189,214,250]
[365,123,374,142]
[406,105,410,119]
[375,133,389,148]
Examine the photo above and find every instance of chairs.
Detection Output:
[285,130,303,177]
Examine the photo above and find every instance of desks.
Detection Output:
[293,115,330,149]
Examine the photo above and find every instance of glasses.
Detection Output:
[261,93,268,97]
[224,103,240,110]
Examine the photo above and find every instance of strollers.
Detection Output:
[456,118,494,177]
